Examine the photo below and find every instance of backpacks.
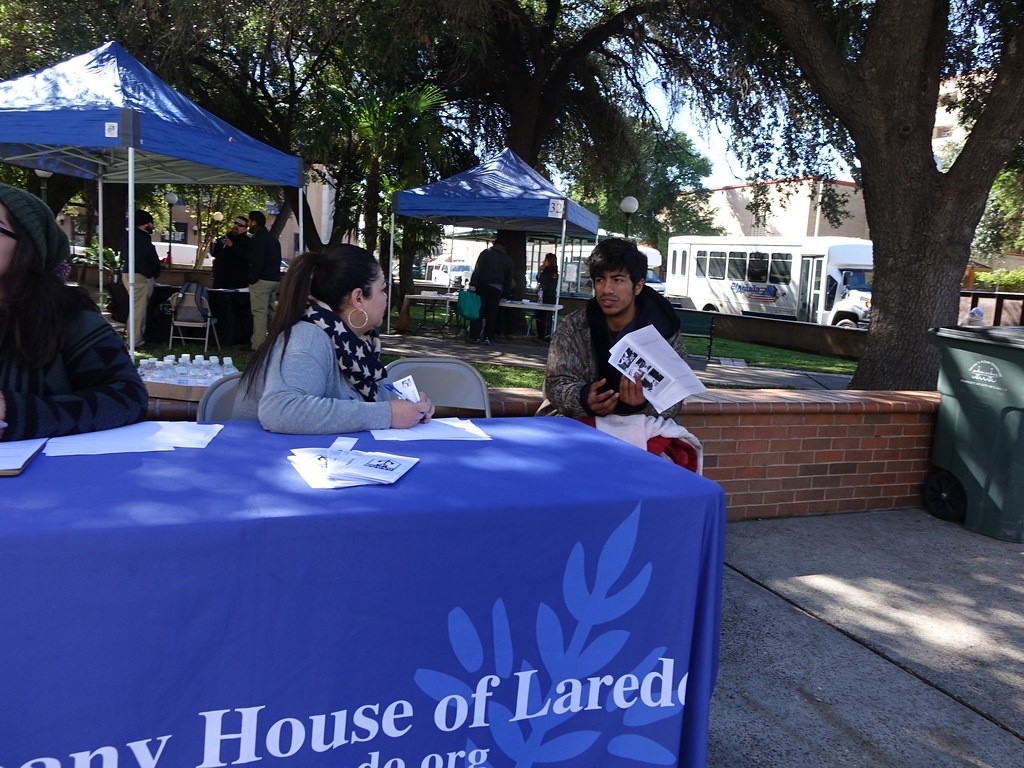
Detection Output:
[172,281,210,322]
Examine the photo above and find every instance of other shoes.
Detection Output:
[464,338,480,344]
[244,344,255,352]
[135,344,154,351]
[483,336,492,346]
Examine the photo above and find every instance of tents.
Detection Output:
[387,148,599,335]
[0,41,305,363]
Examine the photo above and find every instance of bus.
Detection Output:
[525,243,666,299]
[663,233,873,330]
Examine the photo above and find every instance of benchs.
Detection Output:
[675,312,717,361]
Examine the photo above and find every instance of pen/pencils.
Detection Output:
[383,384,431,415]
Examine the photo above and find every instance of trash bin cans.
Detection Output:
[921,325,1024,544]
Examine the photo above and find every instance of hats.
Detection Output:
[0,181,71,285]
[133,210,152,226]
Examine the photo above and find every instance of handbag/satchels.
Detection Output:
[456,288,481,320]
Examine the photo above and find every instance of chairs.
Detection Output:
[524,291,561,337]
[168,292,222,354]
[419,288,441,321]
[196,371,244,422]
[378,357,489,421]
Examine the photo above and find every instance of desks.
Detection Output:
[107,278,252,344]
[497,298,565,352]
[403,292,468,342]
[0,410,736,768]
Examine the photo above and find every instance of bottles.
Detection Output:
[537,288,543,305]
[136,353,239,386]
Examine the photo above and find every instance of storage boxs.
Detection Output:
[137,375,206,420]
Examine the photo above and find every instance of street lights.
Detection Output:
[33,168,55,204]
[212,211,225,239]
[619,195,638,239]
[163,191,180,269]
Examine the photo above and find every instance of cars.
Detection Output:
[150,240,213,268]
[390,259,474,288]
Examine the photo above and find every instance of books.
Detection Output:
[287,448,419,489]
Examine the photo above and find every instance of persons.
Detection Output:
[121,208,160,349]
[960,307,985,327]
[619,353,635,366]
[231,243,435,435]
[209,215,251,290]
[533,253,558,344]
[239,211,281,352]
[465,238,513,347]
[537,238,688,419]
[0,185,148,444]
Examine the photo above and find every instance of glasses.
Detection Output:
[232,221,246,229]
